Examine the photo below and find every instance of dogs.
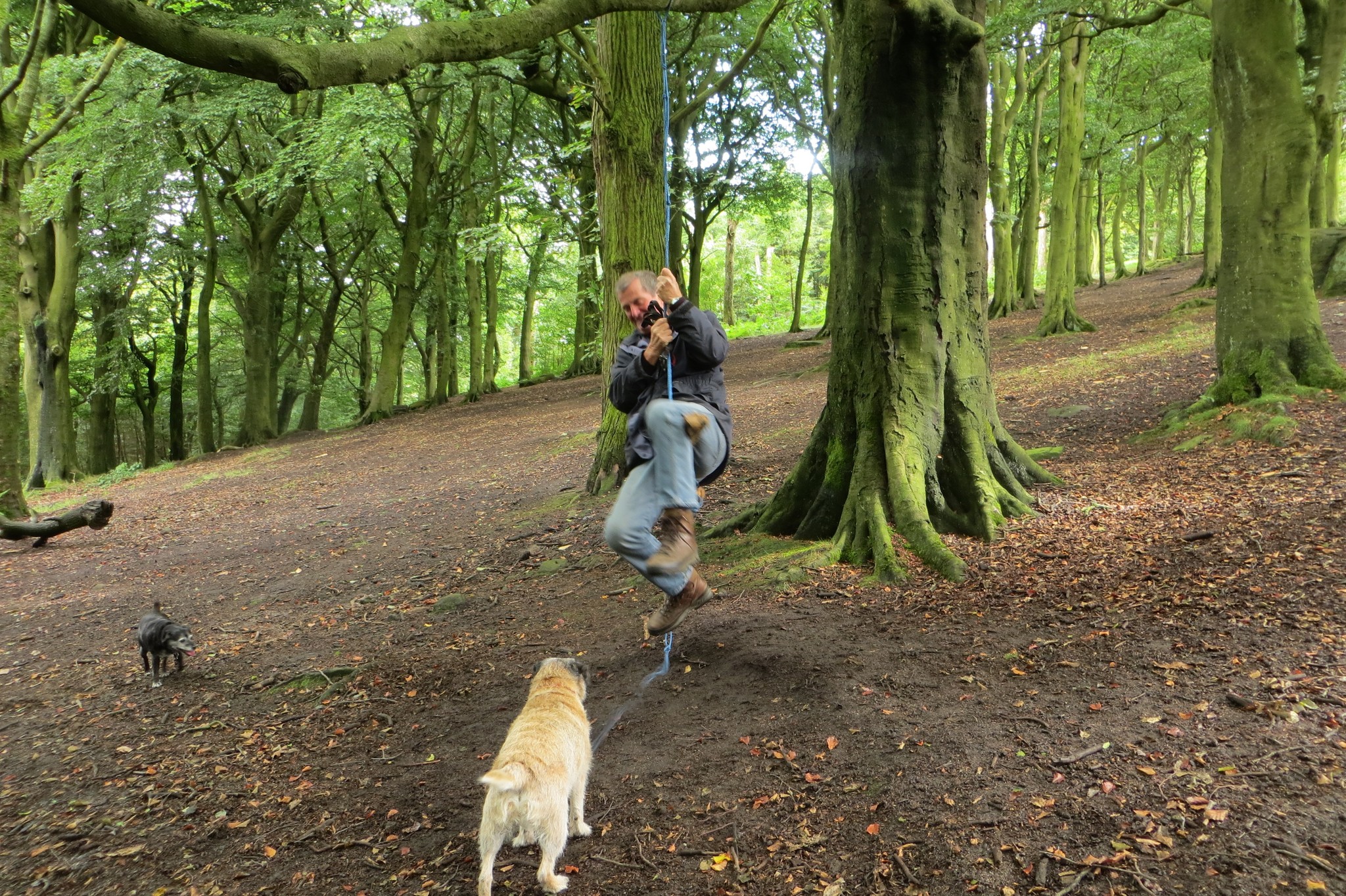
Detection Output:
[472,657,596,895]
[138,601,197,687]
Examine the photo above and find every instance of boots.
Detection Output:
[647,568,713,634]
[645,508,699,574]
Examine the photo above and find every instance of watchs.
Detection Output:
[669,296,681,306]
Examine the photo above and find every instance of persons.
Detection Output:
[602,267,732,633]
